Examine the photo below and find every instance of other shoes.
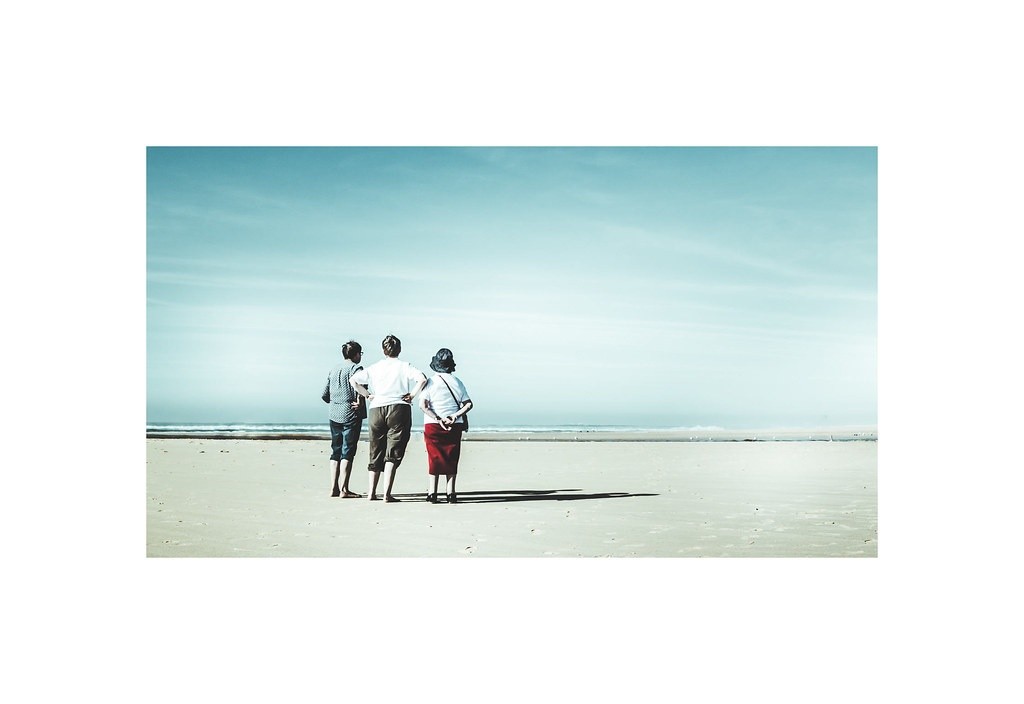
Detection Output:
[425,491,438,503]
[446,494,458,504]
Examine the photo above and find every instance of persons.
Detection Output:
[349,334,427,502]
[320,341,368,498]
[418,348,473,503]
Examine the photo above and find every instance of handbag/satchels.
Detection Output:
[459,407,470,433]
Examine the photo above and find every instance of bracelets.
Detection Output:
[435,416,441,421]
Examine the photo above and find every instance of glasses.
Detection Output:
[356,351,364,357]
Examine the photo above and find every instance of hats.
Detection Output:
[430,348,456,374]
